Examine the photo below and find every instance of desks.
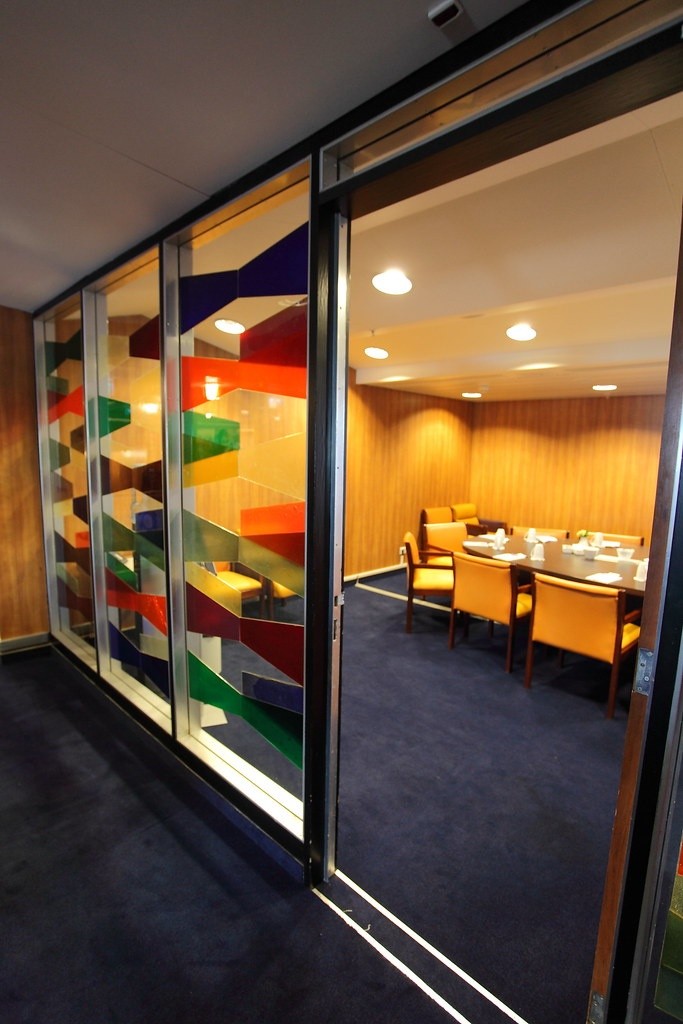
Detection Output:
[463,534,649,599]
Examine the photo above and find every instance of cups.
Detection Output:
[636,563,647,579]
[617,548,635,560]
[526,528,536,542]
[493,528,505,549]
[583,547,598,560]
[592,532,603,545]
[531,543,544,559]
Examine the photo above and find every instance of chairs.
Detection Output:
[449,552,533,672]
[213,560,301,622]
[522,570,641,717]
[421,504,512,600]
[511,527,644,546]
[404,532,454,632]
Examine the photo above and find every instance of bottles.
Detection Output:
[562,532,573,554]
